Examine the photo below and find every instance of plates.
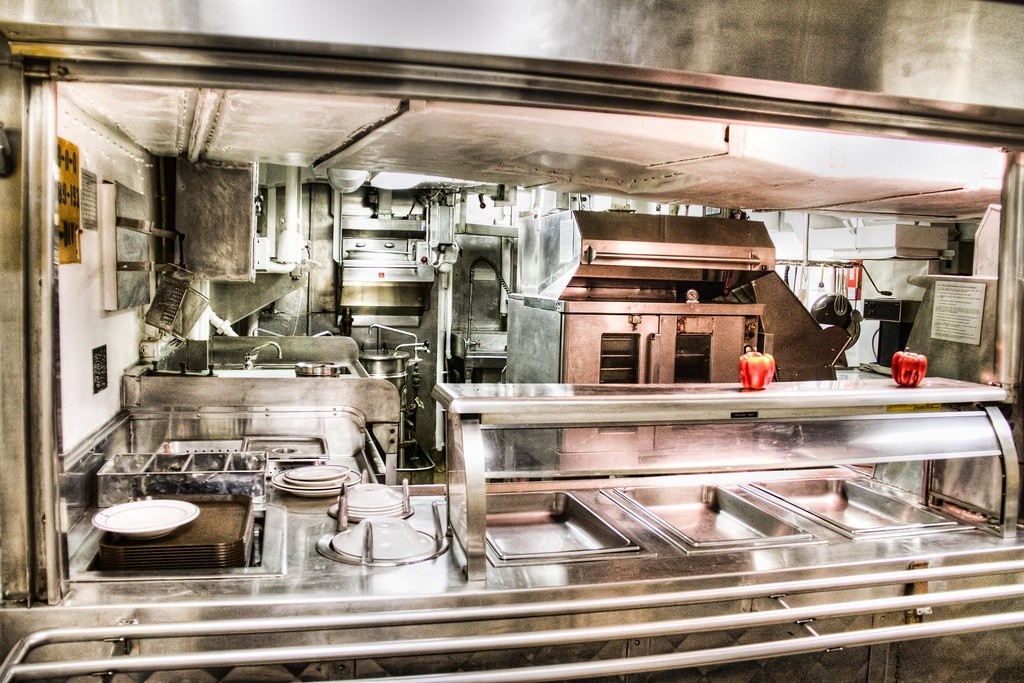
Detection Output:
[90,498,200,542]
[271,463,363,498]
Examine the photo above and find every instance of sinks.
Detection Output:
[482,492,640,560]
[621,484,693,543]
[255,362,351,374]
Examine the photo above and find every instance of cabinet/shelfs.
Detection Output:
[173,157,259,282]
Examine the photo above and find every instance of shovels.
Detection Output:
[851,267,862,322]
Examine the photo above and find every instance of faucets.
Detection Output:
[369,322,417,343]
[245,341,282,368]
[308,330,334,337]
[391,341,430,355]
[252,327,285,336]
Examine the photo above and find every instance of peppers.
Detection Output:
[892,346,927,386]
[738,351,775,390]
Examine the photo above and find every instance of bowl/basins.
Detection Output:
[333,481,429,559]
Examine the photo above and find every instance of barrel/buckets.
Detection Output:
[358,349,410,392]
